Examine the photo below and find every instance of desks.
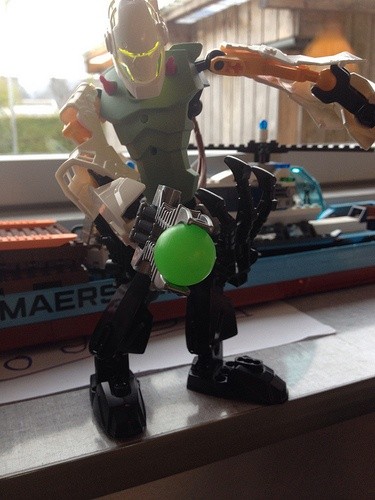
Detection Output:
[0,282,375,500]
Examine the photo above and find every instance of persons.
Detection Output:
[53,1,375,442]
